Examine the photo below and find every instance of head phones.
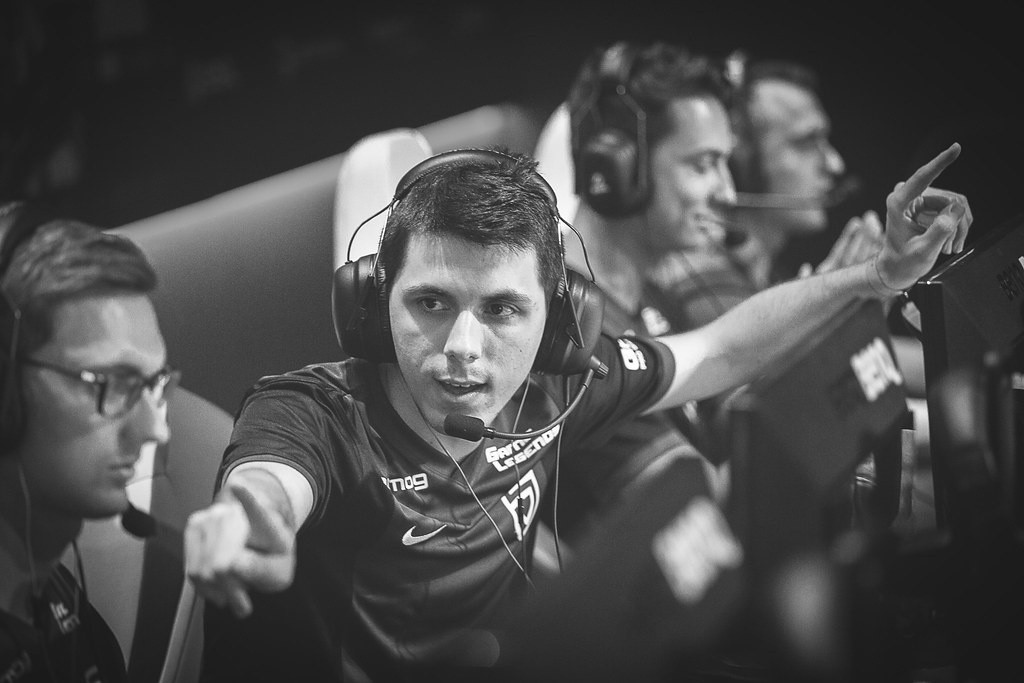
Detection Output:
[719,49,764,202]
[573,42,652,217]
[332,148,609,381]
[0,198,82,446]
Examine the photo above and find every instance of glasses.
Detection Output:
[18,358,182,420]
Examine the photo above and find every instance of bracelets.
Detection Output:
[865,256,904,302]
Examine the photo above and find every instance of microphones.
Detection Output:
[119,502,158,539]
[728,181,862,212]
[444,346,609,443]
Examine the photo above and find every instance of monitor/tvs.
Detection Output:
[719,213,1024,683]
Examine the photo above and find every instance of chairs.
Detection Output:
[331,127,430,350]
[535,103,579,239]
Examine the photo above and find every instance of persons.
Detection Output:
[653,48,881,332]
[183,144,974,682]
[562,40,885,612]
[1,194,176,682]
